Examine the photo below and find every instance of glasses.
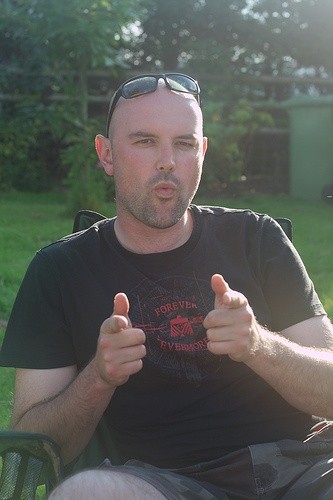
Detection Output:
[106,71,201,139]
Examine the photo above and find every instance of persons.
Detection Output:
[0,70,331,500]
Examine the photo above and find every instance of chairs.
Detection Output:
[1,209,294,500]
